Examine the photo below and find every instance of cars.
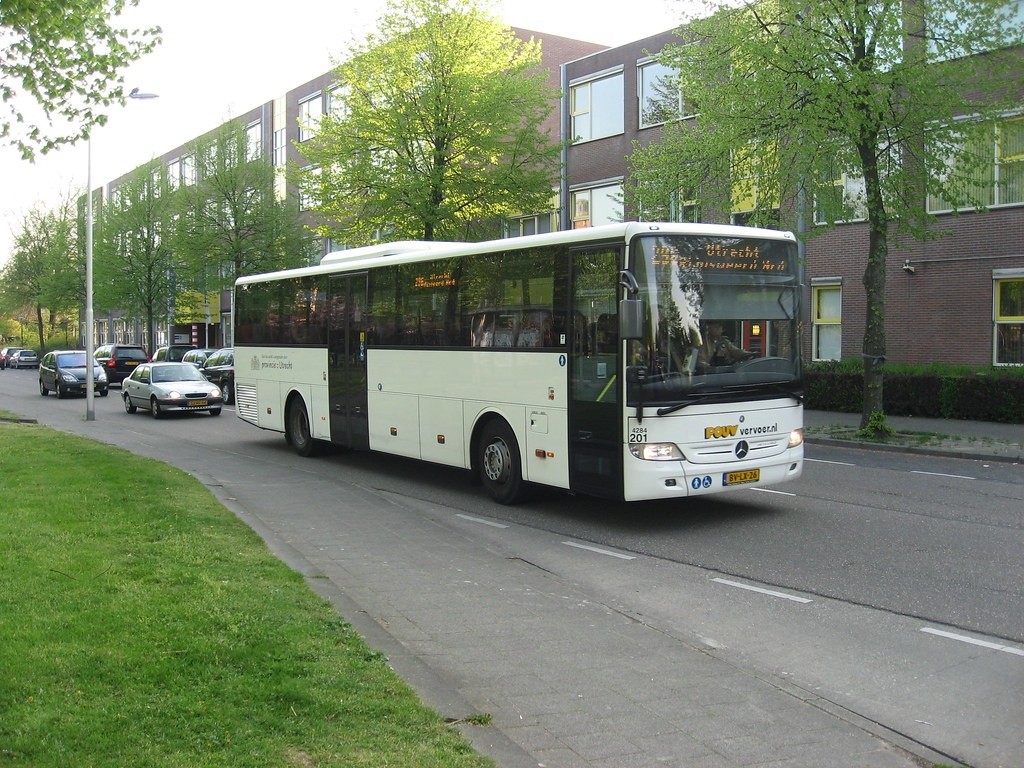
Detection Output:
[181,349,219,368]
[148,345,198,362]
[9,350,39,369]
[197,348,235,405]
[121,362,224,420]
[0,350,6,370]
[39,351,109,399]
[1,347,24,368]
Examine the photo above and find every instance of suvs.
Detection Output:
[92,343,150,386]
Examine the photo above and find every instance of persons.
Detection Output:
[692,321,752,374]
[642,304,668,348]
[575,311,608,352]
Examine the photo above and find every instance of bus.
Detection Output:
[461,305,590,349]
[231,221,804,505]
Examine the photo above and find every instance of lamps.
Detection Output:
[903,266,915,274]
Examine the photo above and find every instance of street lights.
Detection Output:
[86,93,159,420]
[19,315,24,347]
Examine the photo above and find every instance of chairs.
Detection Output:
[165,369,174,376]
